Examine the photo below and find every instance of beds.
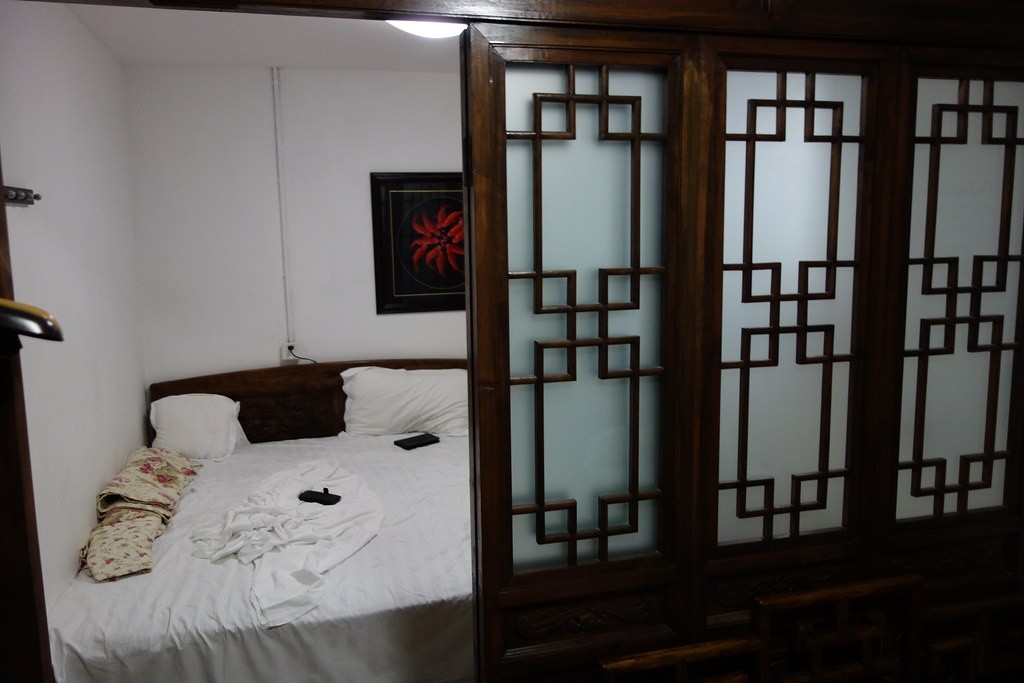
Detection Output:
[51,360,476,683]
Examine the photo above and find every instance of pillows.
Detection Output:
[338,367,468,438]
[150,394,251,461]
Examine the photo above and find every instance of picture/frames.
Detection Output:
[371,171,465,315]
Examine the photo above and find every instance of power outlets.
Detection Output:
[282,344,299,360]
